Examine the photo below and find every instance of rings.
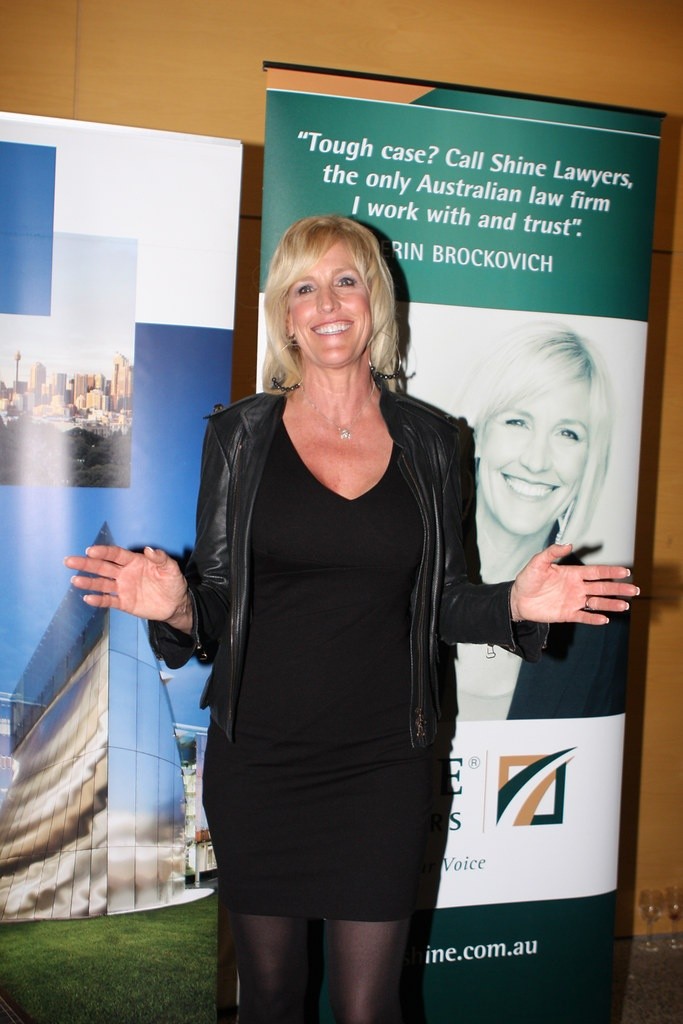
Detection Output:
[584,596,592,608]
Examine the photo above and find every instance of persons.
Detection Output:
[454,316,630,719]
[65,213,642,1023]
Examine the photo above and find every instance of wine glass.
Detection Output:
[665,885,683,951]
[638,888,665,952]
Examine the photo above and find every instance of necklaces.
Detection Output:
[301,380,376,439]
